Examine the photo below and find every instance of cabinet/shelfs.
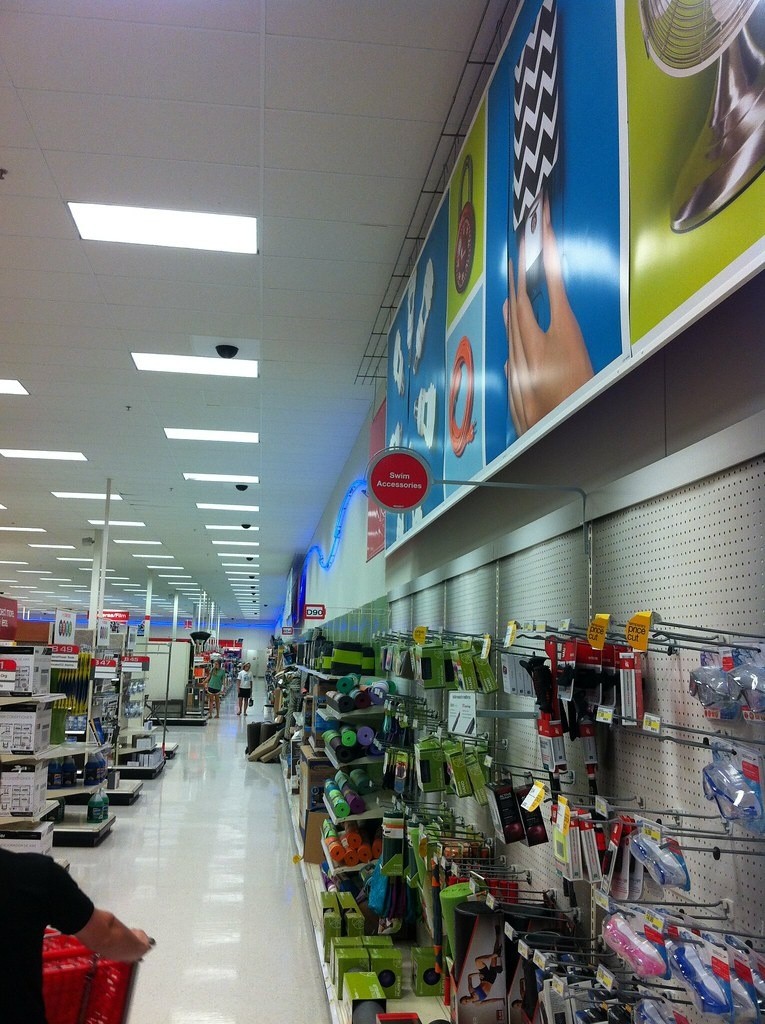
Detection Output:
[287,660,400,881]
[119,721,168,779]
[0,691,69,856]
[43,737,117,841]
[146,644,242,726]
[104,748,144,804]
[265,653,283,708]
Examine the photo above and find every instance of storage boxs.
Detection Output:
[0,703,51,756]
[272,661,339,867]
[0,646,52,696]
[406,625,679,903]
[319,890,450,1024]
[0,821,55,856]
[40,927,132,1023]
[0,761,48,816]
[128,731,162,768]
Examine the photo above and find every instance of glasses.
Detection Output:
[702,764,758,822]
[629,833,678,885]
[602,911,765,1024]
[688,663,765,713]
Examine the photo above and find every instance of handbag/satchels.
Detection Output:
[249,691,253,706]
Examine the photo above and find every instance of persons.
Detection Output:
[202,660,226,718]
[459,924,541,1024]
[237,662,253,716]
[502,189,596,438]
[0,847,152,1024]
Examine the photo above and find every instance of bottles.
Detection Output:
[86,787,110,823]
[48,758,62,789]
[84,752,107,786]
[46,796,66,824]
[62,755,78,787]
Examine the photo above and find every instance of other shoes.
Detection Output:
[237,712,242,715]
[244,713,247,716]
[208,715,212,718]
[214,715,219,718]
